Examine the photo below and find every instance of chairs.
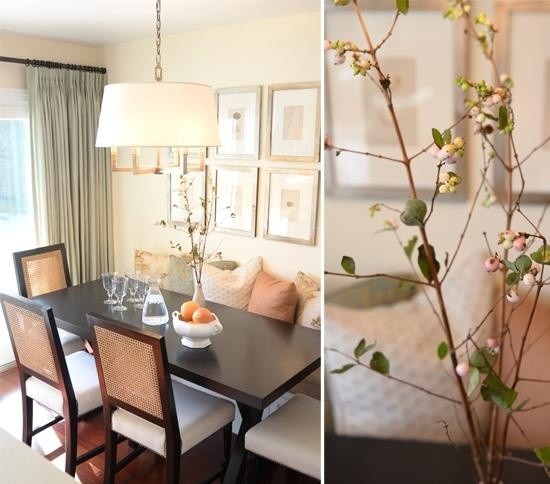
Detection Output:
[0,243,321,484]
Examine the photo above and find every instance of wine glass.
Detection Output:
[103,303,140,323]
[100,270,149,312]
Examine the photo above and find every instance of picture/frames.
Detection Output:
[169,81,321,247]
[322,0,471,201]
[492,0,550,205]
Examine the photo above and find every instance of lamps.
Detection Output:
[95,0,222,175]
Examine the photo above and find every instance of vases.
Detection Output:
[192,284,205,307]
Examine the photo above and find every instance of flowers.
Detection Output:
[153,173,239,284]
[323,0,550,484]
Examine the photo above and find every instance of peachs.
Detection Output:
[192,308,213,323]
[180,301,199,320]
[188,320,193,323]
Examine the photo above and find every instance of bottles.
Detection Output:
[142,278,169,325]
[142,322,170,335]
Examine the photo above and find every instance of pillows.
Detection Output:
[495,288,550,451]
[323,251,493,444]
